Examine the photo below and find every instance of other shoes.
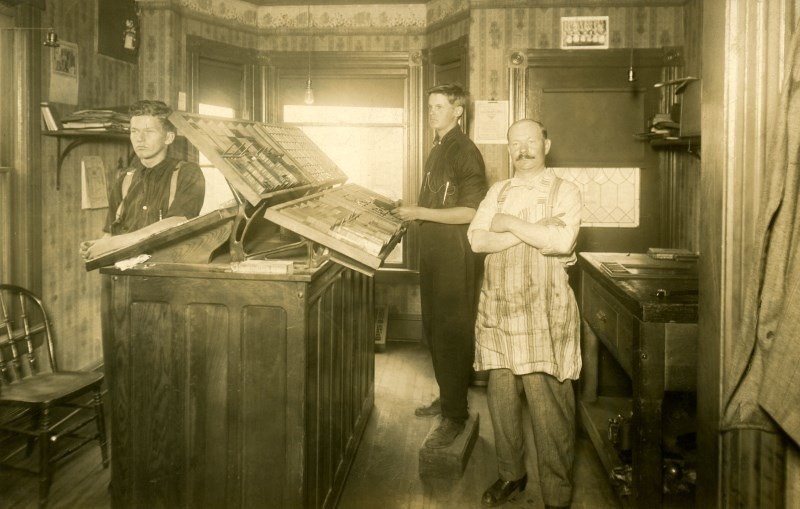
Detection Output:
[426,415,464,449]
[414,397,441,417]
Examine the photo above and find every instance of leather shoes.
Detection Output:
[482,471,528,505]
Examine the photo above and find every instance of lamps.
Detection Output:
[4,26,60,48]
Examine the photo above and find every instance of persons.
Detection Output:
[466,118,583,509]
[392,83,488,450]
[80,99,206,396]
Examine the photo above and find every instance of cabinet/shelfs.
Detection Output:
[577,252,698,509]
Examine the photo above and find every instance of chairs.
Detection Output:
[0,283,110,508]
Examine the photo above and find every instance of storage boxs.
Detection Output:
[419,413,480,479]
[169,110,409,271]
